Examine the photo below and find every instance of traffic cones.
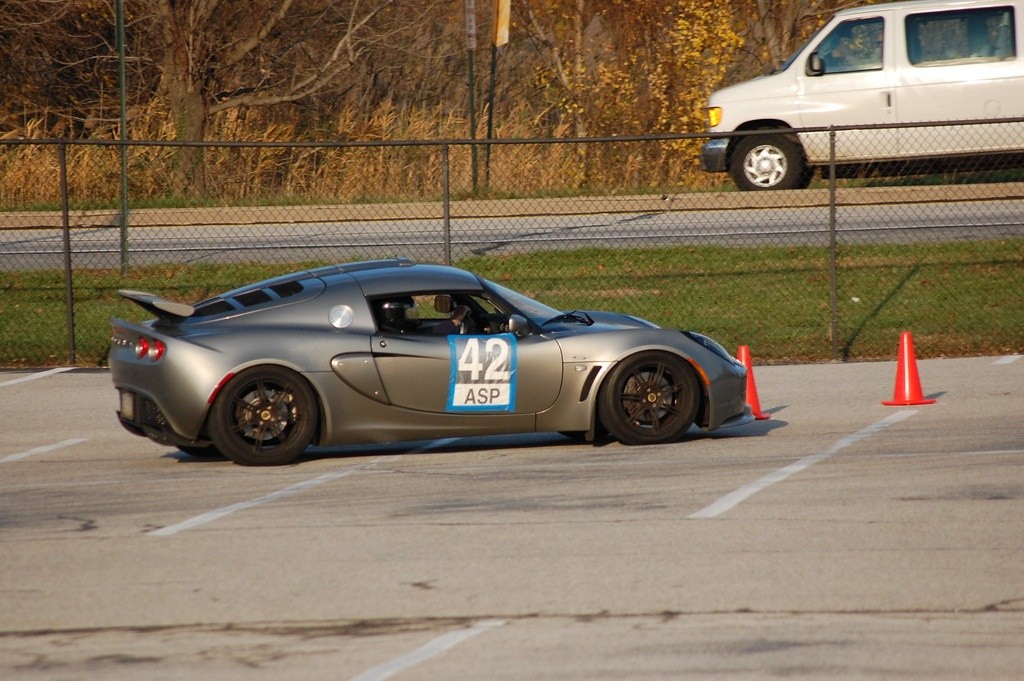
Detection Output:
[880,329,939,406]
[735,345,772,421]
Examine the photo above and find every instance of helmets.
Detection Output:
[378,294,423,331]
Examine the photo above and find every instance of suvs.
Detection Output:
[696,0,1024,192]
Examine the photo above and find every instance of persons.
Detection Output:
[374,296,472,335]
[832,30,885,65]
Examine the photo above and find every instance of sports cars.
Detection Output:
[108,256,757,467]
